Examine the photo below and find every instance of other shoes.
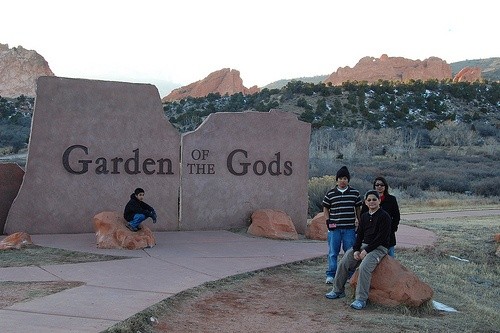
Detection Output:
[325,291,346,299]
[126,222,141,232]
[326,276,334,284]
[350,300,367,310]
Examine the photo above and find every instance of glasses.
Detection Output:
[375,183,385,186]
[367,197,378,202]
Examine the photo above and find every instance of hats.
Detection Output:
[336,166,350,183]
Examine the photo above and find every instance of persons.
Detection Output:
[325,190,397,310]
[123,188,157,232]
[322,166,362,284]
[373,177,400,258]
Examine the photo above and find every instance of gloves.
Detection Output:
[152,211,157,218]
[152,215,157,223]
